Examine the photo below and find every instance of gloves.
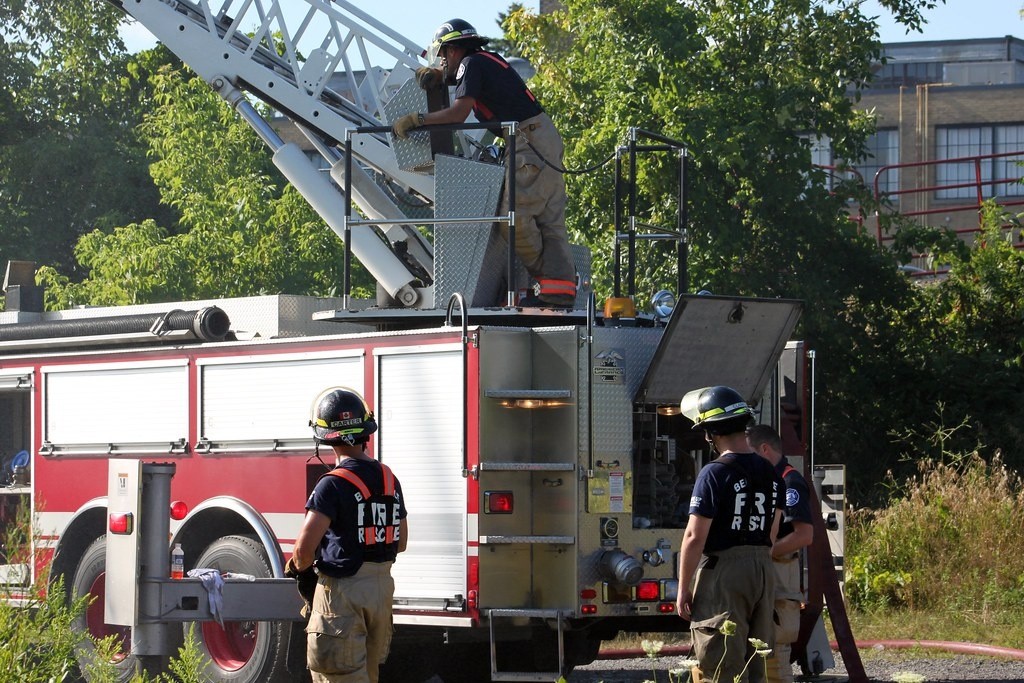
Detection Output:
[391,111,422,140]
[415,67,443,91]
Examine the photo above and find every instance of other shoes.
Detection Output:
[519,297,573,313]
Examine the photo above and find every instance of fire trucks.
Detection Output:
[0,0,848,683]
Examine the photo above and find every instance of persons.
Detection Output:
[748,424,815,683]
[394,18,577,307]
[676,387,786,683]
[284,386,408,682]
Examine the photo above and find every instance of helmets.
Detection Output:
[427,18,490,66]
[690,386,754,428]
[307,386,377,447]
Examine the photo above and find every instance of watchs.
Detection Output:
[417,112,424,125]
